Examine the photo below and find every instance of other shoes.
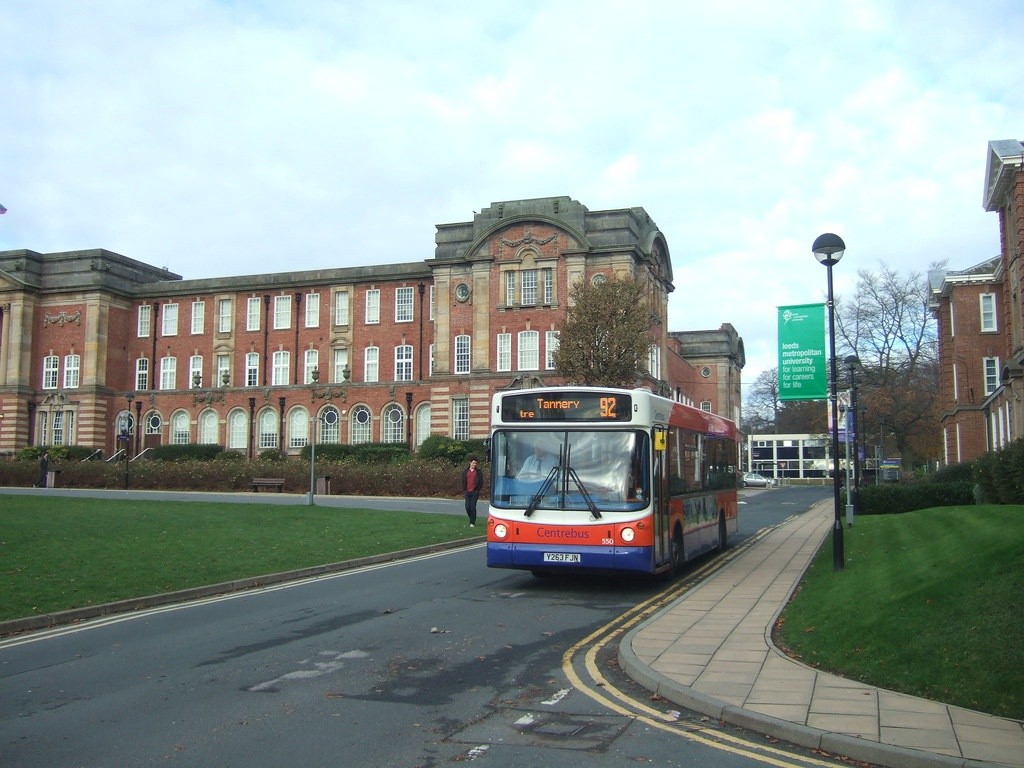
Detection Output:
[469,524,474,528]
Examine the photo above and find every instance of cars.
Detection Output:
[710,468,741,479]
[742,474,775,487]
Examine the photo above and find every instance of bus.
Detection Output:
[486,387,738,577]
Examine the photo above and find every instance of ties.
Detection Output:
[536,459,543,478]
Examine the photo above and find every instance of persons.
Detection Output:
[461,458,483,529]
[515,437,558,481]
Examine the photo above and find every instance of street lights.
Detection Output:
[859,405,868,470]
[812,233,846,573]
[310,416,319,493]
[843,355,861,488]
[124,392,135,490]
